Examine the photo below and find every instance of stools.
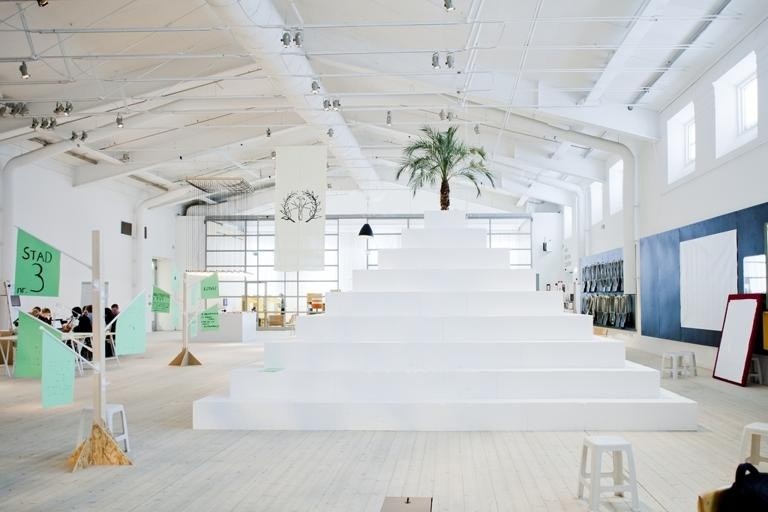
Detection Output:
[738,422,768,473]
[658,351,697,378]
[77,404,129,451]
[747,357,763,384]
[576,434,640,512]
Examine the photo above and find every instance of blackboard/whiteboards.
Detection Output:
[712,293,763,387]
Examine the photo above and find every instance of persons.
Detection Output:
[13,303,120,362]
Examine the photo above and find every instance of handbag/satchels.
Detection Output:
[717,463,767,512]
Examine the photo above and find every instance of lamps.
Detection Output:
[64,102,74,116]
[444,0,456,13]
[19,61,31,79]
[359,199,374,237]
[116,117,123,128]
[445,52,455,71]
[312,81,341,111]
[293,28,305,46]
[280,29,291,48]
[432,52,441,70]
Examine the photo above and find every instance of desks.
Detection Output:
[0,332,122,378]
